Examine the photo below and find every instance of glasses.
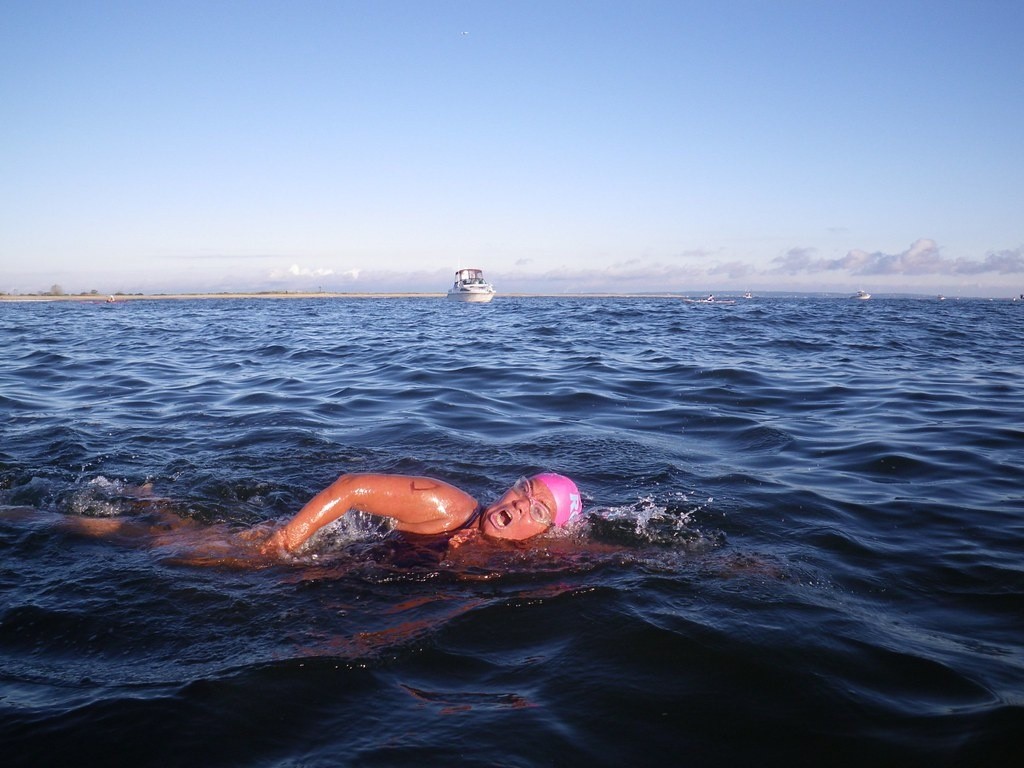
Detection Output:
[514,474,554,528]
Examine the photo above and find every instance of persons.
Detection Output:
[0,461,780,592]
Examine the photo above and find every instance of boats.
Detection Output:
[936,294,946,300]
[1019,294,1024,300]
[849,290,871,300]
[447,269,496,302]
[743,292,753,299]
[683,299,735,304]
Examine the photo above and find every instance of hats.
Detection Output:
[531,472,583,527]
